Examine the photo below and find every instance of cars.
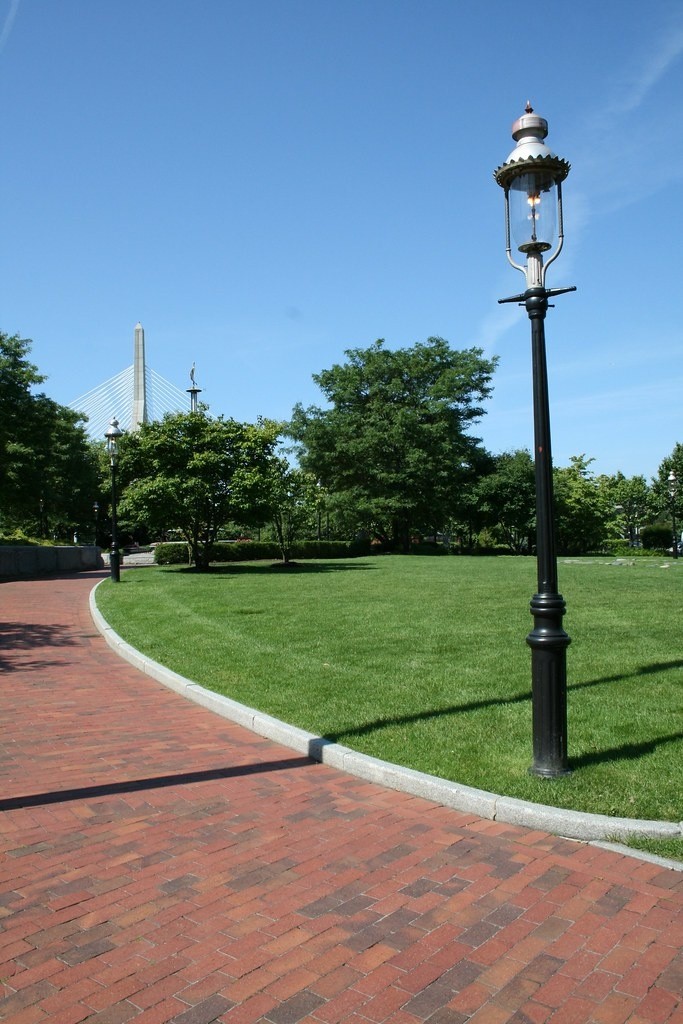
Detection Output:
[668,541,683,557]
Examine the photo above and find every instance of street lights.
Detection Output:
[91,500,99,545]
[667,470,678,560]
[493,99,577,778]
[103,416,125,582]
